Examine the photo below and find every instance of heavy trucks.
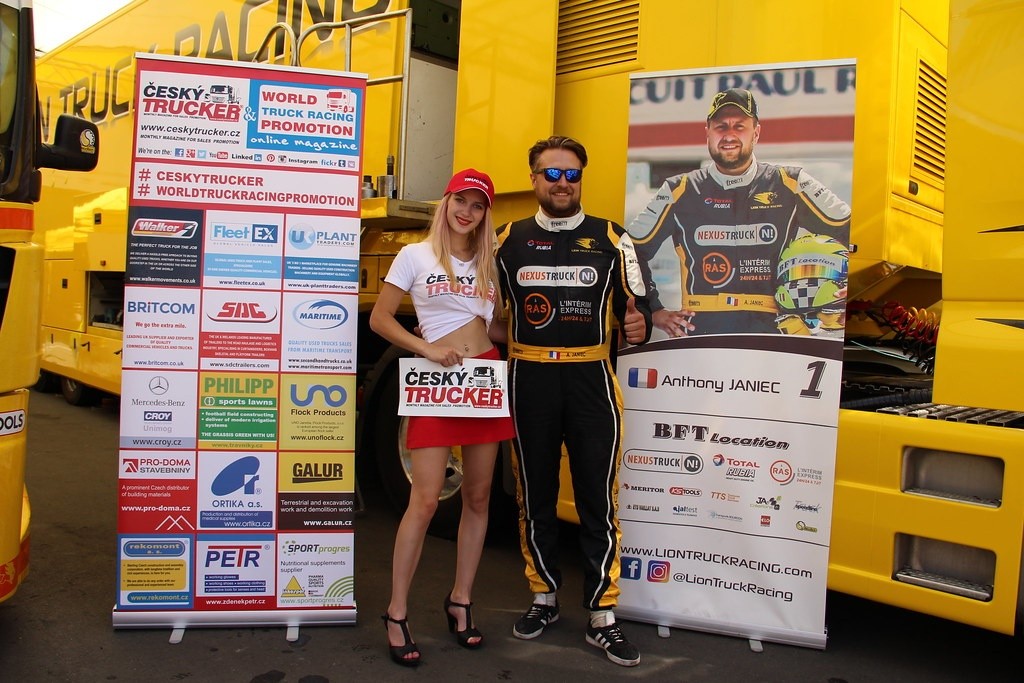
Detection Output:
[35,2,1022,639]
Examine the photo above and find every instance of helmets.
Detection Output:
[775,235,849,335]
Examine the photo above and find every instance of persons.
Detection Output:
[412,135,655,669]
[368,169,510,669]
[625,86,851,336]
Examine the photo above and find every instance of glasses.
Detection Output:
[535,168,582,184]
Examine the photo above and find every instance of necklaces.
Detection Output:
[449,248,473,266]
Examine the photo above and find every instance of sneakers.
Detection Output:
[512,593,558,640]
[585,611,640,666]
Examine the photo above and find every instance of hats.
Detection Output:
[445,168,494,210]
[708,89,760,121]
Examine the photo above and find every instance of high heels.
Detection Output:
[443,592,483,651]
[381,612,420,668]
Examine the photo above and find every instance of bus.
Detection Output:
[0,0,98,604]
